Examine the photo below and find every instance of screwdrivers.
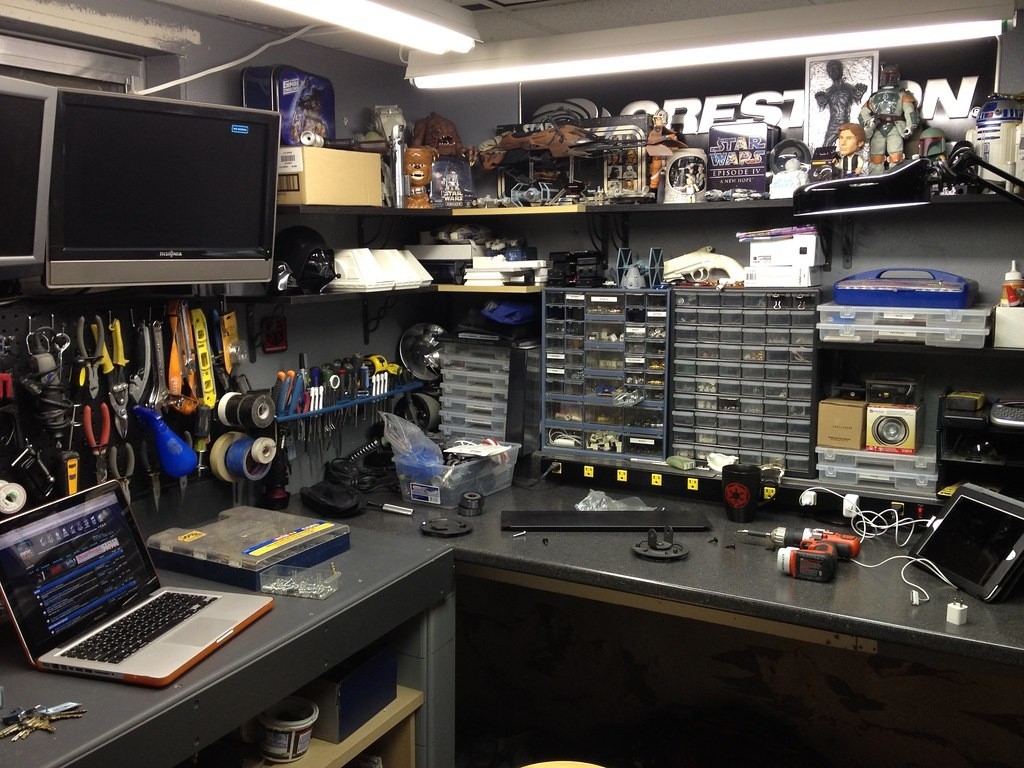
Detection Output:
[195,405,211,478]
[307,353,370,477]
[371,372,389,425]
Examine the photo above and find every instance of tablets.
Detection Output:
[906,486,1024,602]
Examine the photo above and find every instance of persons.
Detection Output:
[649,110,679,193]
[608,134,623,166]
[670,161,705,195]
[401,146,434,209]
[815,60,868,149]
[830,122,866,180]
[861,65,918,176]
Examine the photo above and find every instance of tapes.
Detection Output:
[209,392,278,483]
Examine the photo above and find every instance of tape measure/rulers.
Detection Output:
[364,353,387,379]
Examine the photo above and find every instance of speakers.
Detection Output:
[871,415,910,448]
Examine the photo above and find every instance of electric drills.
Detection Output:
[737,526,862,583]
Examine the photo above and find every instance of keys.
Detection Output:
[0,701,87,741]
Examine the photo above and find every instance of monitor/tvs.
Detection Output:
[0,76,56,281]
[42,85,281,288]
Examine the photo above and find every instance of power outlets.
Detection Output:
[260,315,288,354]
[617,470,627,481]
[552,463,562,474]
[763,487,776,500]
[651,474,662,486]
[584,466,594,477]
[687,478,699,490]
[891,502,904,516]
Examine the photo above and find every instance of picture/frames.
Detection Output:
[799,49,880,159]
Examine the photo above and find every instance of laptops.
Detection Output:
[0,480,275,686]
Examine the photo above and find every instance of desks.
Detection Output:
[263,381,423,445]
[0,493,459,768]
[241,683,425,768]
[286,482,1024,680]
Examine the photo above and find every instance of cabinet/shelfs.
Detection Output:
[936,383,1024,506]
[225,206,453,363]
[438,192,1024,357]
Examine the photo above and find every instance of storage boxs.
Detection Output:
[146,503,352,591]
[259,564,342,602]
[391,436,522,510]
[405,242,485,260]
[992,304,1024,349]
[276,145,383,206]
[706,120,781,192]
[299,641,397,742]
[485,247,537,284]
[240,64,335,144]
[438,233,997,497]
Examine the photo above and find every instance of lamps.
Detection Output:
[254,0,484,55]
[403,0,1024,91]
[791,142,1024,217]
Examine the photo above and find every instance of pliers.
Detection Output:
[75,315,193,512]
[274,369,311,449]
